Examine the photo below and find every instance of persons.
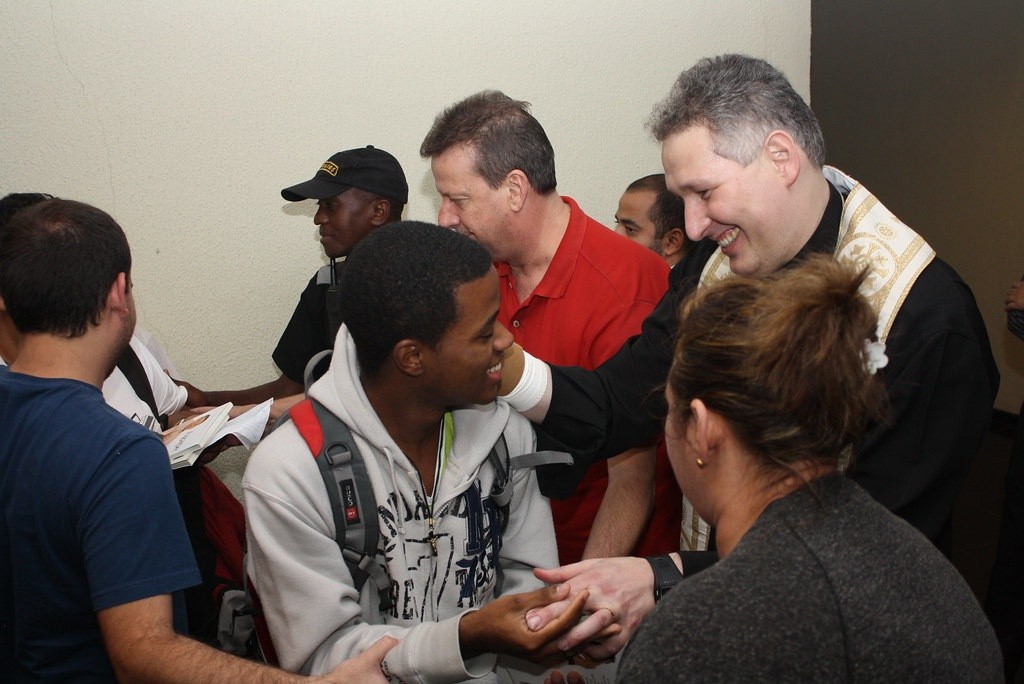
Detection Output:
[163,414,210,446]
[613,174,693,267]
[614,254,1005,684]
[1003,274,1024,341]
[0,200,398,684]
[238,220,590,684]
[525,51,1002,660]
[163,146,410,451]
[191,90,682,566]
[0,192,226,466]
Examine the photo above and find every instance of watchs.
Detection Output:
[645,554,683,605]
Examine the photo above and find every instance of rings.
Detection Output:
[600,607,615,621]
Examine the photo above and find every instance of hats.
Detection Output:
[282,146,408,204]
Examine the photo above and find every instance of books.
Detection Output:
[161,401,233,471]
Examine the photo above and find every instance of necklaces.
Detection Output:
[405,416,441,557]
